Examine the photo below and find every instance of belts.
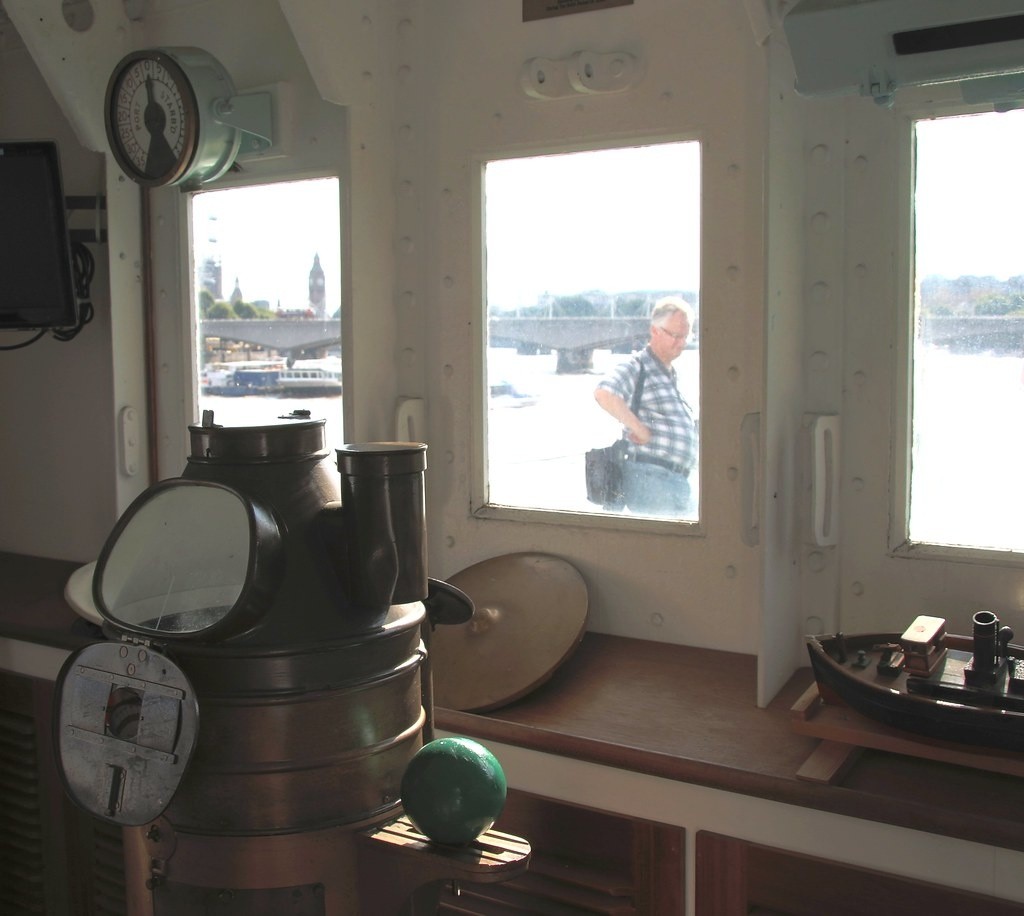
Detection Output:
[626,451,691,479]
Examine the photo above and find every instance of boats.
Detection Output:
[488,379,542,408]
[807,609,1023,756]
[199,357,343,397]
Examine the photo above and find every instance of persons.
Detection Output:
[594,304,698,516]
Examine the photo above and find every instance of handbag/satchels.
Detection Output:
[585,440,633,513]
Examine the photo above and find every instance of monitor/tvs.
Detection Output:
[0,139,79,332]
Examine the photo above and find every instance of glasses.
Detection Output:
[660,327,689,341]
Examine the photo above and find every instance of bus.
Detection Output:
[277,307,318,319]
